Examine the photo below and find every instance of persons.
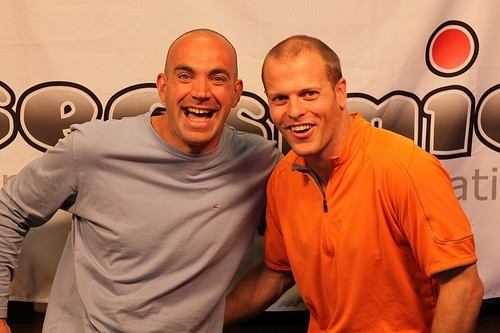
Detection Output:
[226,35,484,333]
[0,28,287,333]
